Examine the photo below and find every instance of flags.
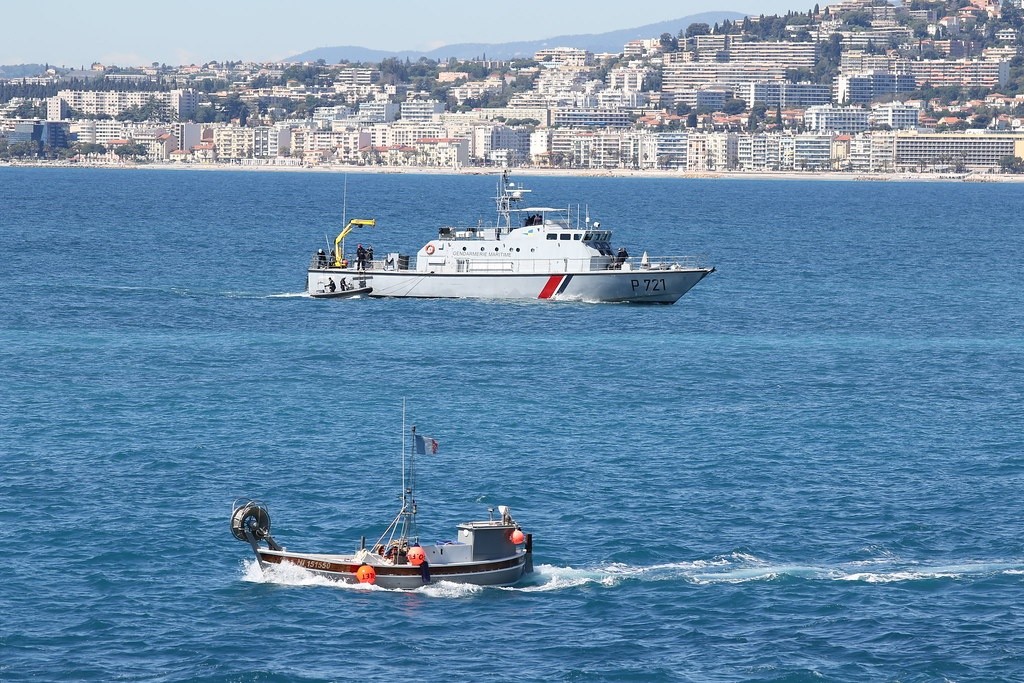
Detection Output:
[415,435,438,454]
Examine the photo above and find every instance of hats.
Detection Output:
[358,244,362,249]
[318,249,323,253]
[342,276,346,278]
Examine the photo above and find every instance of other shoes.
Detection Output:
[357,267,360,270]
[362,267,366,271]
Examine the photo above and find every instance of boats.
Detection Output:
[306,169,716,305]
[310,287,373,298]
[229,396,534,590]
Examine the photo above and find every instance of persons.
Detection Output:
[316,249,326,269]
[525,213,542,226]
[339,277,347,291]
[356,244,368,270]
[616,247,629,269]
[366,245,373,264]
[325,278,336,292]
